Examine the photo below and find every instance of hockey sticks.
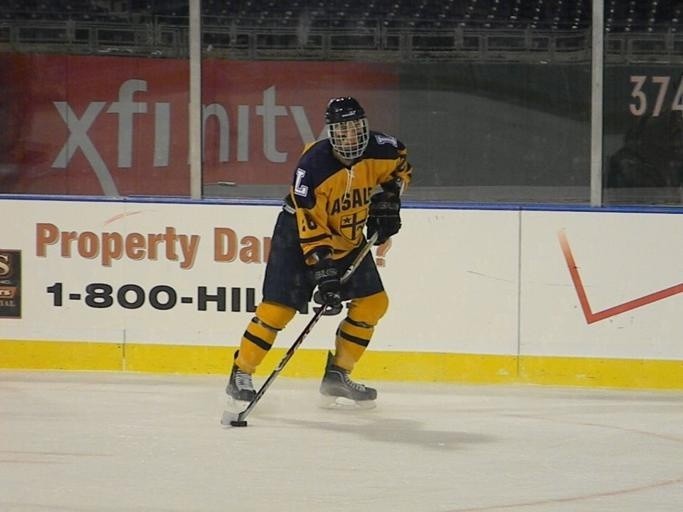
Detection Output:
[220,232,379,425]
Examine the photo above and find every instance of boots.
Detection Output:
[225,349,255,400]
[318,349,377,401]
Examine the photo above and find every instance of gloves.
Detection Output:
[367,191,401,243]
[316,263,340,307]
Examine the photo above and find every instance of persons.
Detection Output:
[225,95,413,402]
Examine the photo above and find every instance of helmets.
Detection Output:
[325,96,370,159]
